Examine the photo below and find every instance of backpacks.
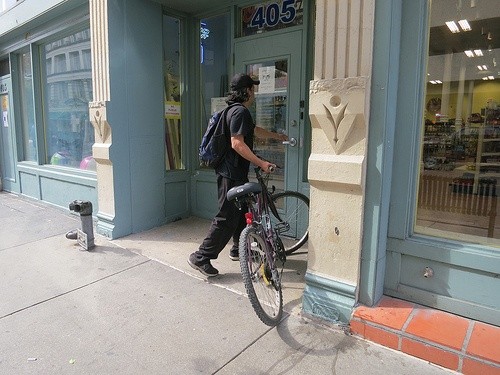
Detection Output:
[198,103,246,168]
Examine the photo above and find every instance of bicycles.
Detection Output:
[225,153,310,327]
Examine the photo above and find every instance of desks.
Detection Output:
[424,98,500,196]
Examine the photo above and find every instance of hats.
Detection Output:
[230,73,261,90]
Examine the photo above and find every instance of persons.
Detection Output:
[187,72,288,276]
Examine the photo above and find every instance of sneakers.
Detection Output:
[187,254,219,276]
[229,250,260,261]
